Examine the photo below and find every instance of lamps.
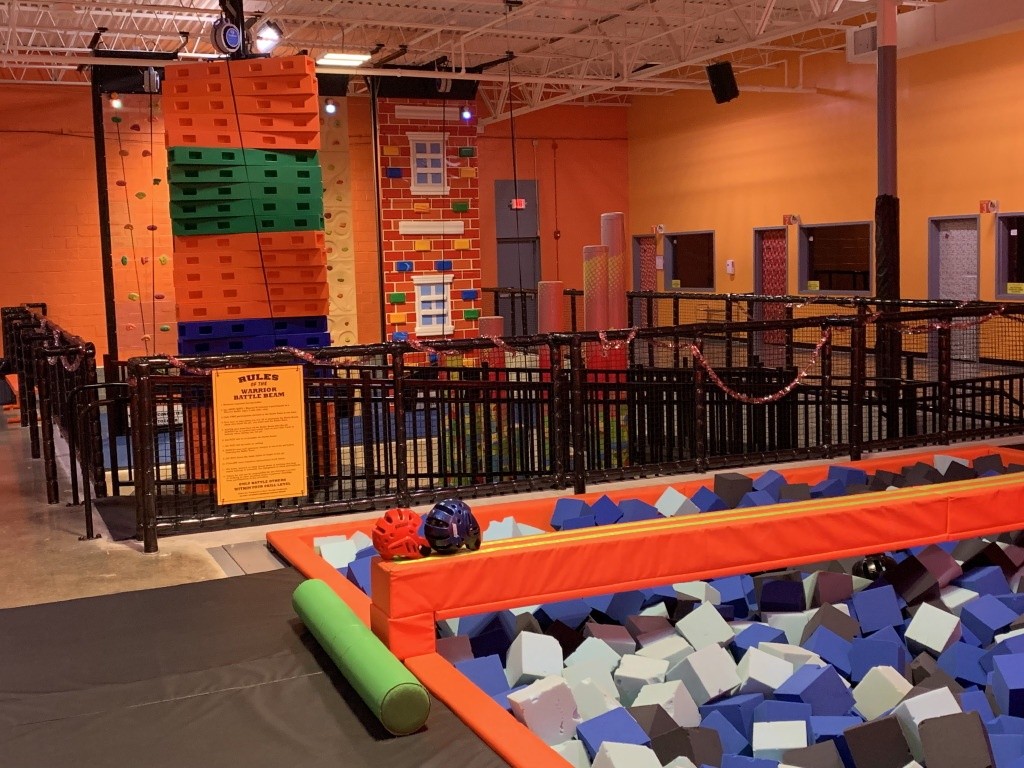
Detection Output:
[705,59,740,103]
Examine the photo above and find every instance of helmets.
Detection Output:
[372,508,431,560]
[423,498,481,553]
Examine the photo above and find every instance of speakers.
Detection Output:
[706,61,739,103]
